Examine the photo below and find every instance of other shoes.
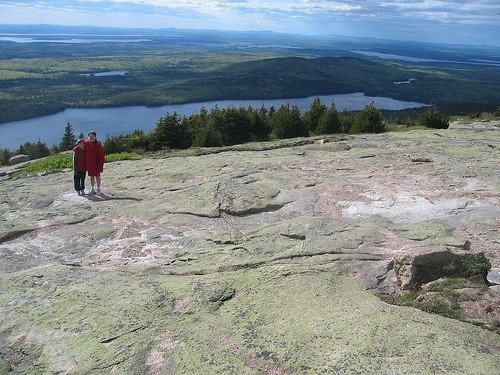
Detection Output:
[82,190,85,194]
[88,189,95,195]
[77,192,81,196]
[97,189,101,195]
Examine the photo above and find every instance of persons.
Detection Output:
[73,131,105,195]
[73,140,104,195]
